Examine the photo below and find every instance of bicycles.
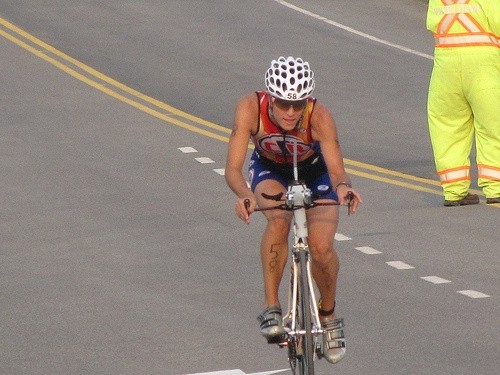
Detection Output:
[245,177,354,375]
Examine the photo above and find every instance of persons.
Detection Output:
[426,0,500,206]
[225,58,362,363]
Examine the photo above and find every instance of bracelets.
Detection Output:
[336,182,352,191]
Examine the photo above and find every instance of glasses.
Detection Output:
[271,96,307,111]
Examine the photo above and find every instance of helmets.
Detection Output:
[264,56,316,100]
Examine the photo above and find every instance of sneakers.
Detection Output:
[443,193,479,206]
[258,306,284,337]
[321,317,346,364]
[486,198,500,203]
